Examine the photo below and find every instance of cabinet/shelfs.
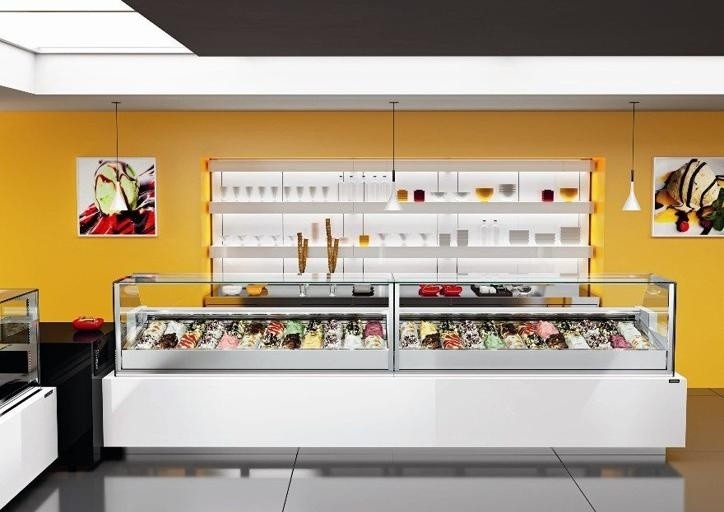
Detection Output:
[114,275,395,373]
[1,288,43,407]
[204,158,599,309]
[394,276,678,376]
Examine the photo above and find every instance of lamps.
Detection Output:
[622,102,642,213]
[385,102,403,212]
[109,102,130,212]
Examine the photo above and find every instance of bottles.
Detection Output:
[413,190,424,202]
[491,219,501,245]
[479,219,489,246]
[397,189,407,202]
[337,175,390,202]
[542,190,554,202]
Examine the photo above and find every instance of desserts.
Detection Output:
[135,318,651,350]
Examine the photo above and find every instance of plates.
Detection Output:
[509,226,581,245]
[420,285,463,296]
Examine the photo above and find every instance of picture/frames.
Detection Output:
[651,156,723,238]
[74,156,158,238]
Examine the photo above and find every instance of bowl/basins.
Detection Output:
[560,188,578,202]
[456,230,468,247]
[246,284,265,296]
[431,184,516,202]
[439,234,450,247]
[222,286,243,295]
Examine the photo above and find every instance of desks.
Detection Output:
[0,322,115,473]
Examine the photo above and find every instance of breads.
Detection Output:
[667,158,720,213]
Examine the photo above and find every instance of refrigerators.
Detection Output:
[113,272,673,379]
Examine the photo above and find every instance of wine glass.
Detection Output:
[375,232,388,246]
[220,234,231,246]
[236,233,248,245]
[218,186,329,202]
[418,231,432,247]
[253,234,265,245]
[287,235,298,246]
[269,233,280,246]
[397,232,411,247]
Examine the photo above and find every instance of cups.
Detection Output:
[359,235,369,246]
